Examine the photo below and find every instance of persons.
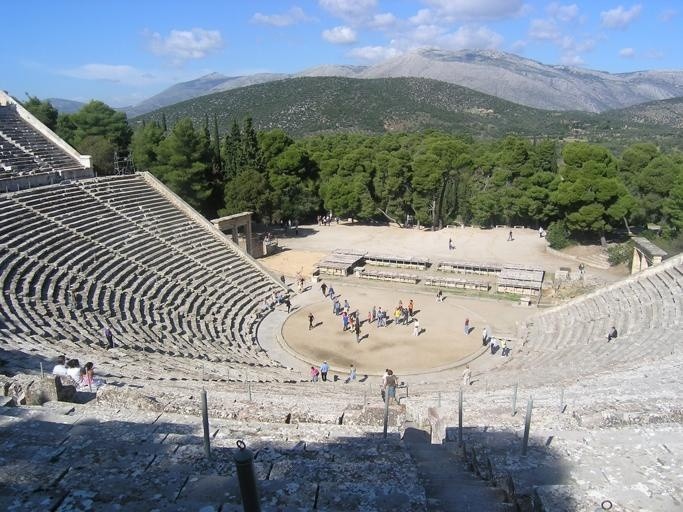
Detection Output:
[349,364,356,381]
[464,317,469,336]
[104,326,113,351]
[491,336,496,354]
[481,327,487,346]
[308,364,319,381]
[317,215,340,227]
[277,266,445,344]
[279,220,299,234]
[320,361,330,381]
[539,226,543,237]
[52,354,95,386]
[607,326,617,343]
[507,230,512,241]
[579,262,586,274]
[501,337,508,356]
[380,368,400,403]
